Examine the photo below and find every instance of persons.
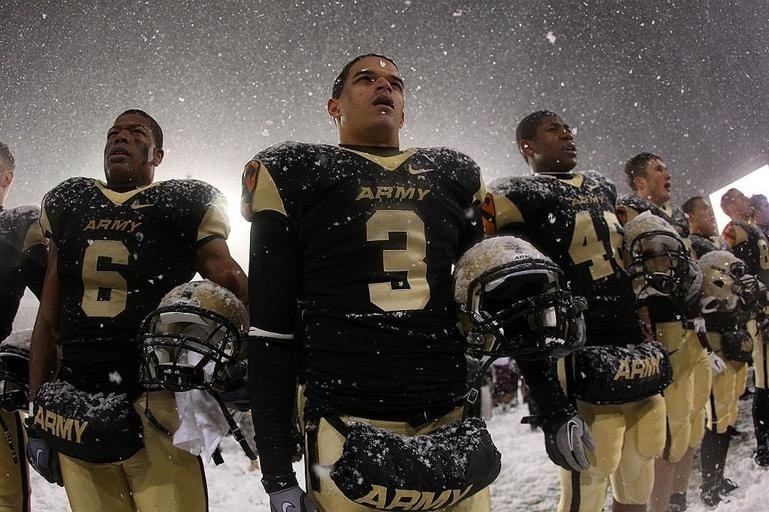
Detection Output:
[29,108,251,511]
[615,152,715,511]
[239,52,534,512]
[679,187,768,505]
[0,142,49,512]
[486,110,674,512]
[236,407,261,478]
[487,347,524,416]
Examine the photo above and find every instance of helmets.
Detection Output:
[0,327,33,413]
[697,250,748,310]
[137,278,250,394]
[622,214,697,293]
[454,235,576,358]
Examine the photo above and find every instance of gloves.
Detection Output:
[263,474,309,512]
[519,402,595,473]
[24,419,60,486]
[703,350,728,375]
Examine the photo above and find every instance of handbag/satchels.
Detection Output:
[564,344,673,406]
[720,329,754,362]
[330,415,502,512]
[31,381,147,465]
